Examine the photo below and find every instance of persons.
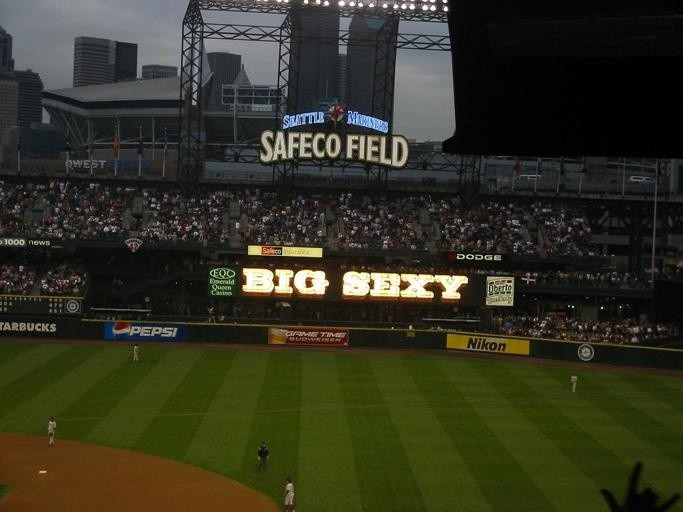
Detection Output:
[257,442,269,473]
[47,418,56,446]
[600,462,681,512]
[132,344,140,361]
[571,374,577,392]
[284,478,296,512]
[0,178,683,348]
